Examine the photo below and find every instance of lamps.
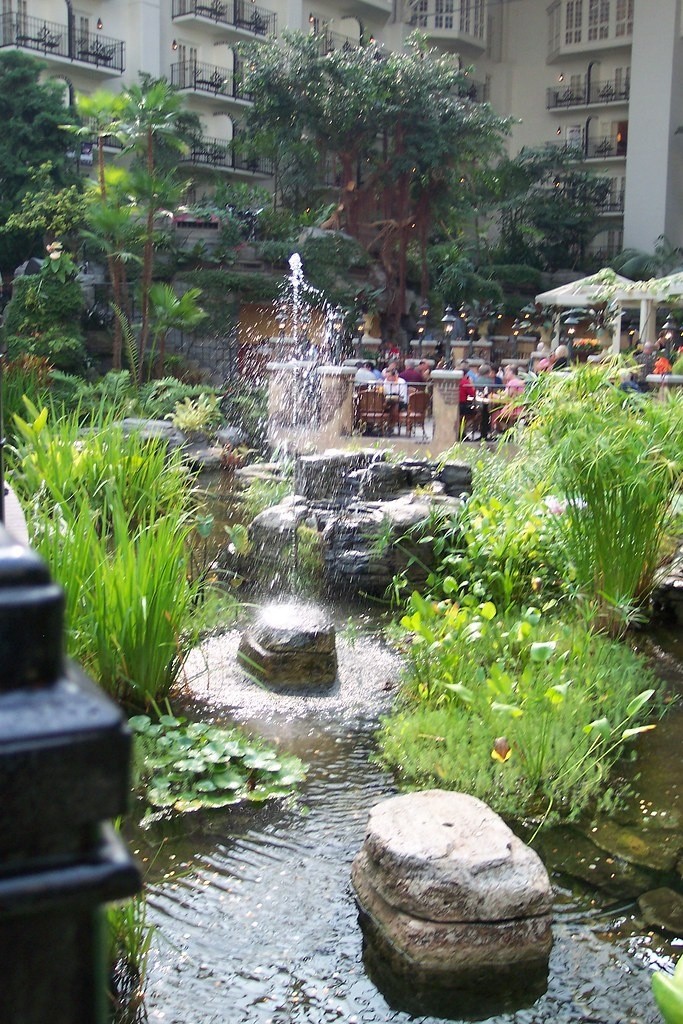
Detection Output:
[309,12,314,23]
[556,127,561,135]
[558,74,563,82]
[555,177,559,188]
[369,35,374,44]
[98,17,103,30]
[172,39,178,50]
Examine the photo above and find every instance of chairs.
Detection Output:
[352,388,429,437]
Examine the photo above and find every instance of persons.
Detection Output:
[339,333,683,442]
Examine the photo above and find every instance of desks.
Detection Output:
[468,397,506,442]
